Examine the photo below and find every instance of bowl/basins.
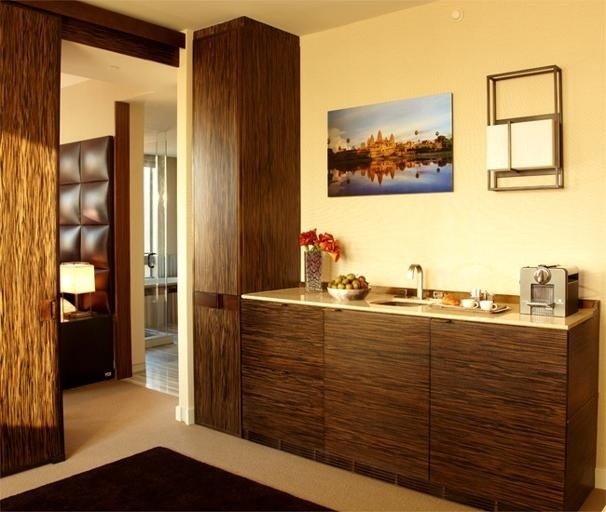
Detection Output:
[327,286,372,302]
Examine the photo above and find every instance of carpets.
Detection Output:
[1,446,338,512]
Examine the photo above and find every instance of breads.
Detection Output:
[442,293,460,306]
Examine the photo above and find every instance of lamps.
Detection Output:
[60,262,95,319]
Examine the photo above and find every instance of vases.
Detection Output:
[304,251,323,292]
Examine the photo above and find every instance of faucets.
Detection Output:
[408,264,424,300]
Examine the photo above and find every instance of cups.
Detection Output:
[471,288,481,298]
[479,300,498,311]
[460,298,479,310]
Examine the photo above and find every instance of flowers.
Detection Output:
[298,228,341,262]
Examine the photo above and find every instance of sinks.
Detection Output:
[366,298,436,307]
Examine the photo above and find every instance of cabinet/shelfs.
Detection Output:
[243,285,600,511]
